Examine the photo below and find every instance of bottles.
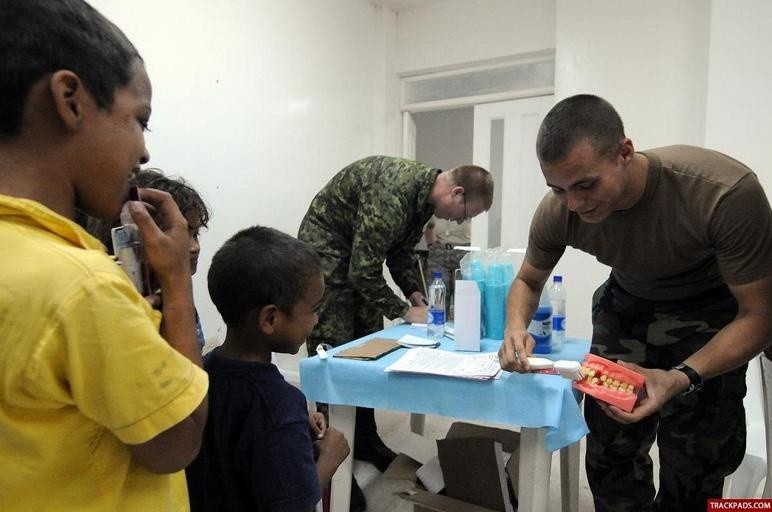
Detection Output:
[427,272,447,340]
[530,274,566,355]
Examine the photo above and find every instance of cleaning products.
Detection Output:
[527,284,554,353]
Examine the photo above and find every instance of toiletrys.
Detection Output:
[465,259,516,340]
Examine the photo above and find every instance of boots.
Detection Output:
[350,473,367,512]
[355,423,397,473]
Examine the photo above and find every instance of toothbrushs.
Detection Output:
[515,355,585,382]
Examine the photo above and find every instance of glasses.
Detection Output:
[464,191,471,220]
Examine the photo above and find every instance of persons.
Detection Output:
[182,222,352,512]
[0,0,212,512]
[296,155,496,509]
[89,169,211,355]
[496,91,772,511]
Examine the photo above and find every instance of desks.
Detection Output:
[299,318,591,512]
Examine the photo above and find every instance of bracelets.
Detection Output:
[671,364,701,399]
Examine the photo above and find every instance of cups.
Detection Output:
[462,261,518,340]
